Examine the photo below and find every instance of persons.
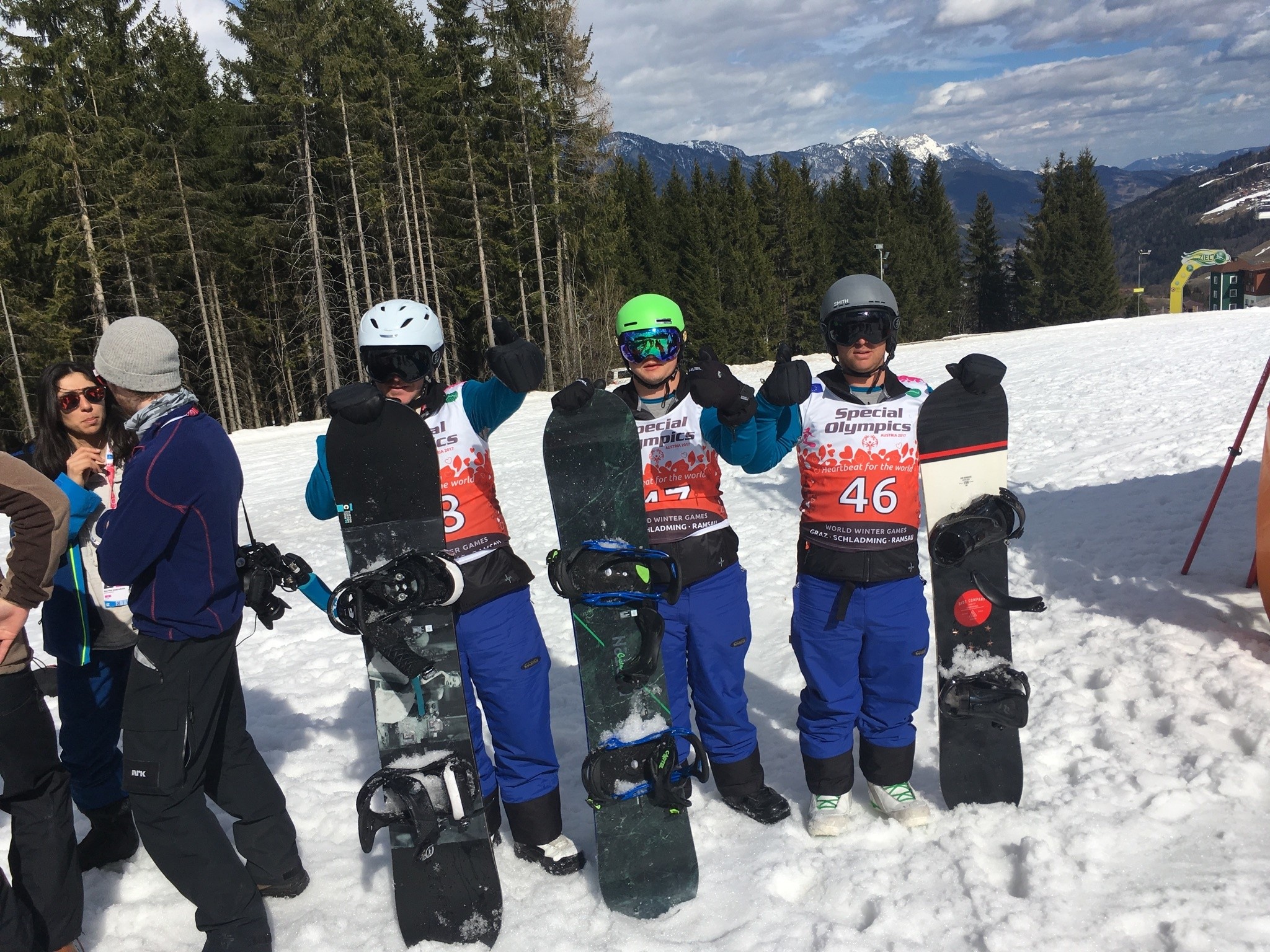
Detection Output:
[0,450,85,952]
[94,317,310,952]
[550,294,791,826]
[306,298,587,877]
[10,360,138,873]
[742,274,1007,837]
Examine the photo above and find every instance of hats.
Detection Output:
[93,316,181,392]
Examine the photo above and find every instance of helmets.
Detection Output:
[616,291,686,373]
[820,273,900,364]
[357,299,443,383]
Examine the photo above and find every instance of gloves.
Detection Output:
[760,344,812,405]
[485,315,544,393]
[685,344,756,418]
[326,382,384,425]
[945,354,1005,393]
[550,376,606,412]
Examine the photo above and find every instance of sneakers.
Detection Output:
[724,789,792,824]
[514,840,587,877]
[868,777,930,825]
[808,790,850,836]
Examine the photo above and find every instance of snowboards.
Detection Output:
[539,386,703,918]
[913,379,1032,808]
[324,394,509,948]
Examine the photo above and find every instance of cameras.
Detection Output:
[238,542,292,630]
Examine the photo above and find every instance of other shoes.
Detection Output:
[256,866,308,897]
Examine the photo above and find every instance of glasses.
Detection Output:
[826,309,894,346]
[54,385,106,414]
[619,327,682,364]
[360,346,433,383]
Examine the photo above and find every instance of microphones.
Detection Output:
[281,555,339,618]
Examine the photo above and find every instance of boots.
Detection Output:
[77,803,140,874]
